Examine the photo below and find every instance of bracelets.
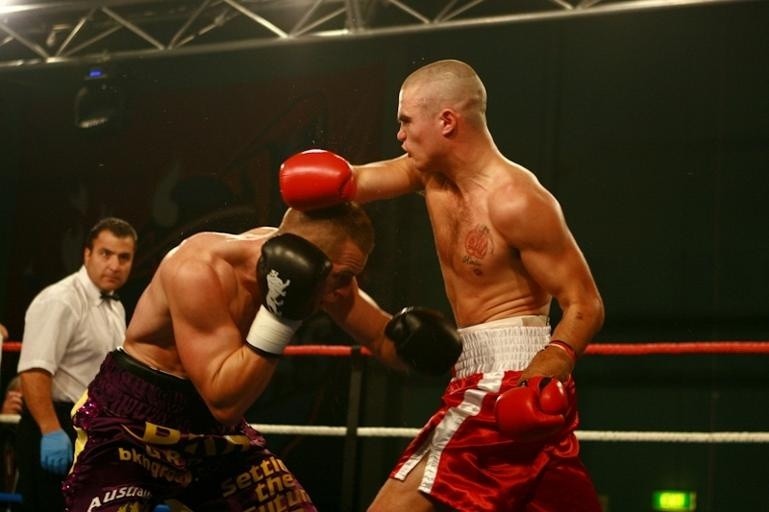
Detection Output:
[244,302,303,360]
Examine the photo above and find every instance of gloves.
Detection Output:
[245,233,333,360]
[281,148,360,213]
[385,305,464,377]
[495,340,578,438]
[40,429,74,477]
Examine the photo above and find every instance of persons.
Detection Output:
[13,217,138,512]
[279,59,605,512]
[0,323,9,364]
[2,375,23,415]
[61,200,463,512]
